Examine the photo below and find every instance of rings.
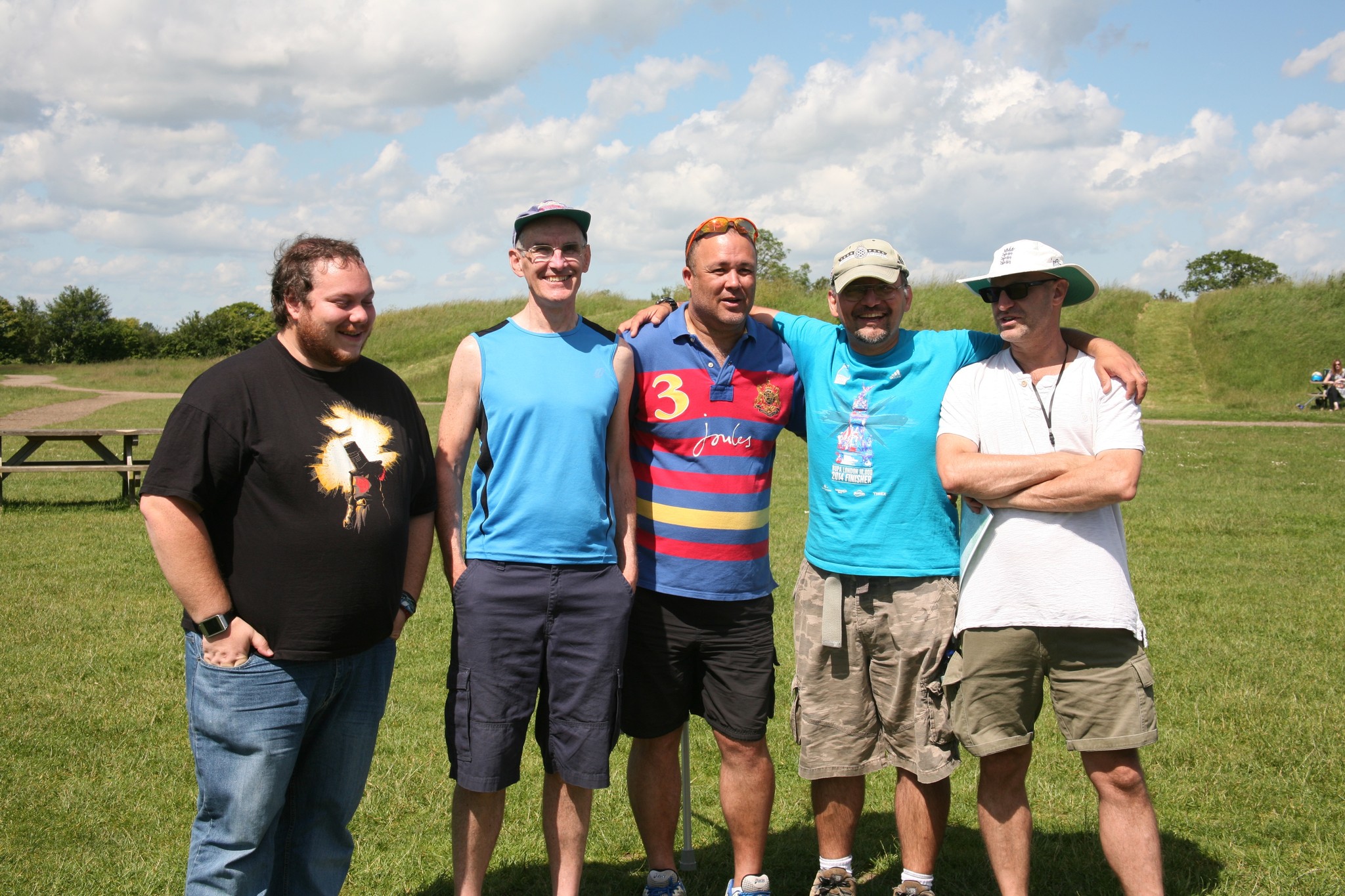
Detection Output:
[1140,372,1145,377]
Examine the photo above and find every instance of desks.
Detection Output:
[0,428,166,512]
[1309,380,1331,409]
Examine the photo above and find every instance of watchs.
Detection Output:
[655,296,679,313]
[192,606,238,638]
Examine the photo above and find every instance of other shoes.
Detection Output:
[893,877,934,896]
[725,873,771,896]
[642,869,686,896]
[810,867,857,896]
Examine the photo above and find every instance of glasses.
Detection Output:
[686,217,759,260]
[844,283,906,301]
[516,243,586,259]
[977,278,1060,304]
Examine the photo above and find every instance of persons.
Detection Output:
[435,198,639,896]
[1323,358,1345,411]
[137,238,438,896]
[616,238,1148,896]
[621,216,808,896]
[936,240,1163,895]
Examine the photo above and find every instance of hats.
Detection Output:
[956,240,1099,308]
[513,199,591,249]
[831,238,909,295]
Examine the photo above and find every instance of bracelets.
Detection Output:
[400,589,416,620]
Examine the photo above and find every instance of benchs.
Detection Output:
[1306,392,1327,399]
[0,458,150,474]
